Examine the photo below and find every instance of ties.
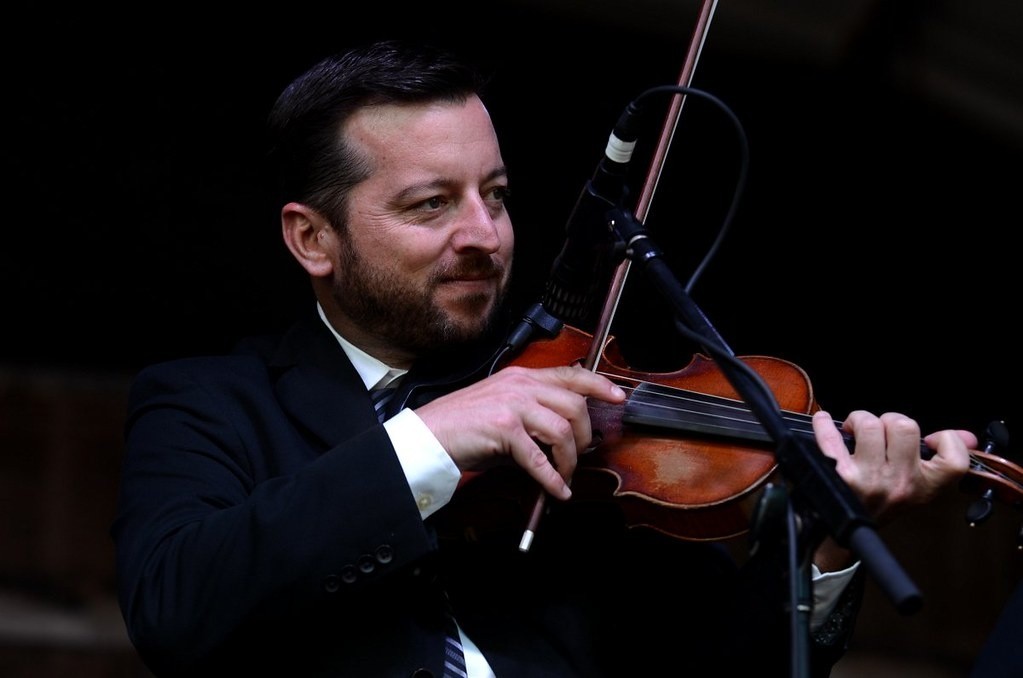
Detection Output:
[368,386,469,678]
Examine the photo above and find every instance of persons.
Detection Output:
[114,39,978,678]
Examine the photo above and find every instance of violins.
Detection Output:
[451,321,1023,543]
